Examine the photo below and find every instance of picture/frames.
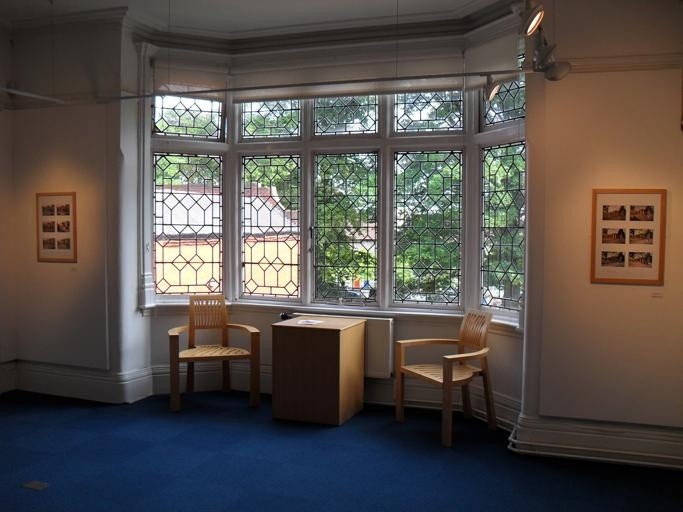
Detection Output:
[587,187,667,287]
[35,191,77,264]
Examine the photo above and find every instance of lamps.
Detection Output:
[479,71,502,102]
[516,4,569,82]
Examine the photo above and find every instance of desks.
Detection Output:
[269,315,365,424]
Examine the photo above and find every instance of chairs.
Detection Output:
[395,308,496,442]
[168,294,259,410]
